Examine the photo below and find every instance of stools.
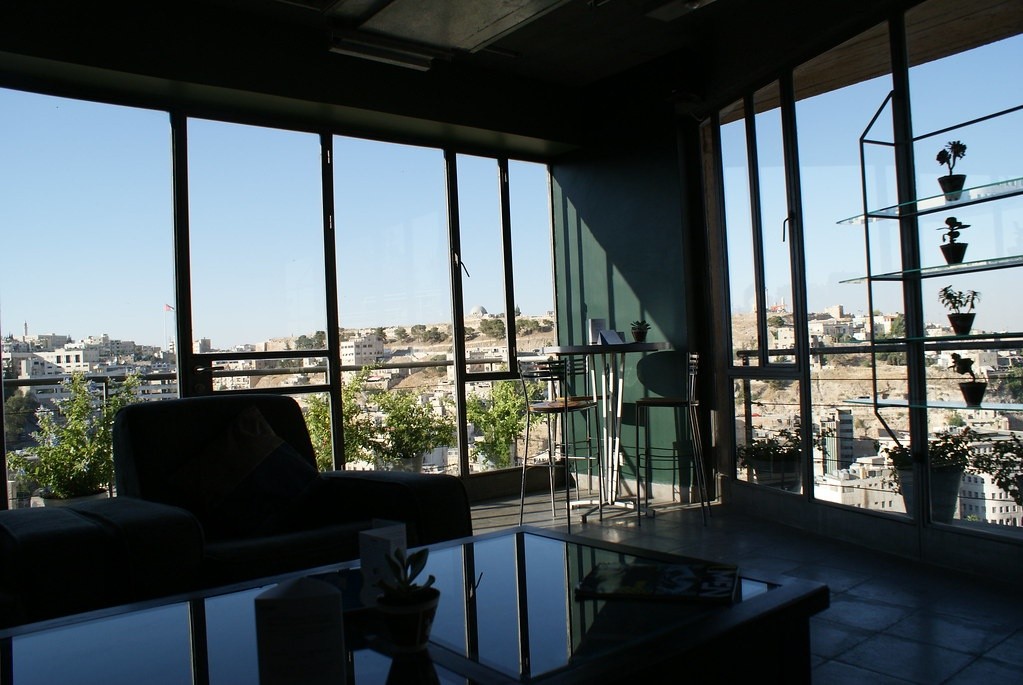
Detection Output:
[0,507,114,628]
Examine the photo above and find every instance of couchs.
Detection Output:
[69,393,473,598]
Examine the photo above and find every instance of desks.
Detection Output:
[0,525,829,685]
[553,338,671,524]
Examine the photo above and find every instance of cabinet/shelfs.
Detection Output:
[837,90,1023,447]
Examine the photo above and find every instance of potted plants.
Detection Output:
[937,217,971,265]
[940,284,979,335]
[467,376,546,471]
[366,387,452,472]
[947,353,988,406]
[735,413,835,492]
[5,367,148,507]
[630,320,651,342]
[883,426,982,521]
[936,140,967,200]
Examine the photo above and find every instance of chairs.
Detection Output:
[635,352,712,528]
[513,347,597,525]
